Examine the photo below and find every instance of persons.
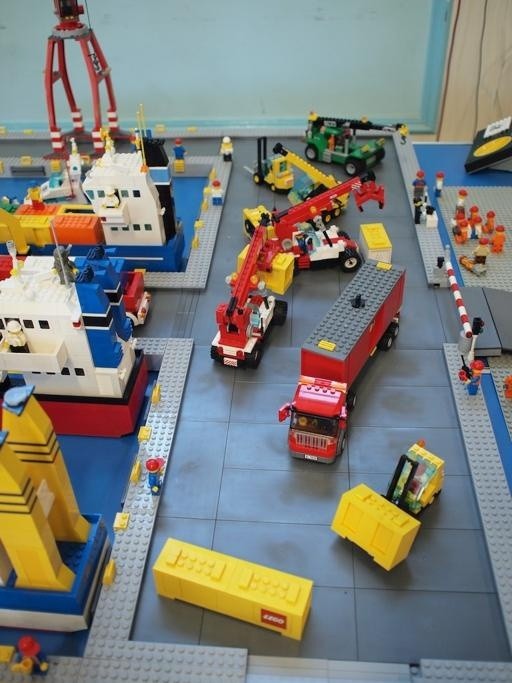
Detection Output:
[172,139,187,160]
[412,171,426,203]
[295,231,313,256]
[461,360,484,395]
[435,173,507,265]
